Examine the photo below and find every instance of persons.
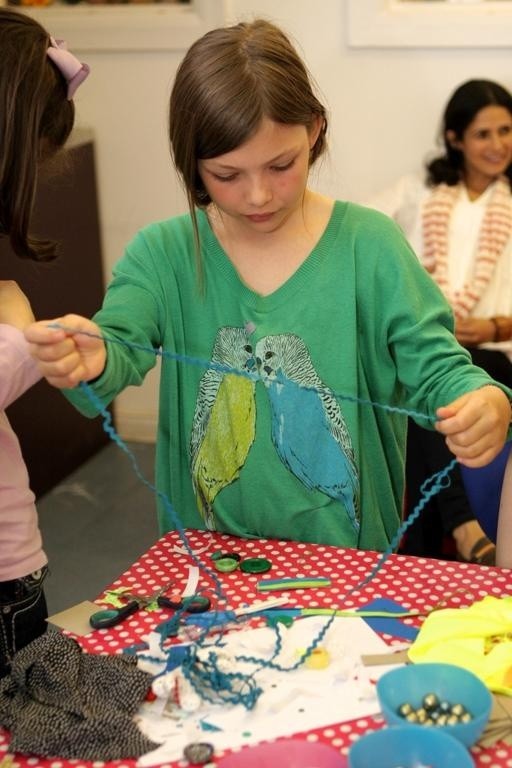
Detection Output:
[382,80,512,574]
[24,17,512,558]
[0,9,91,678]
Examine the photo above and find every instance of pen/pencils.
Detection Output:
[256,577,331,589]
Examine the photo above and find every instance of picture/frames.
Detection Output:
[339,1,511,58]
[0,0,238,57]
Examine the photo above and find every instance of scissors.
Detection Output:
[89,581,211,628]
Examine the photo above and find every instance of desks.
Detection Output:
[1,525,512,768]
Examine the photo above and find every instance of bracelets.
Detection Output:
[489,316,502,342]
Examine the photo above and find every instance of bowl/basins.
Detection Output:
[374,662,490,750]
[345,724,477,767]
